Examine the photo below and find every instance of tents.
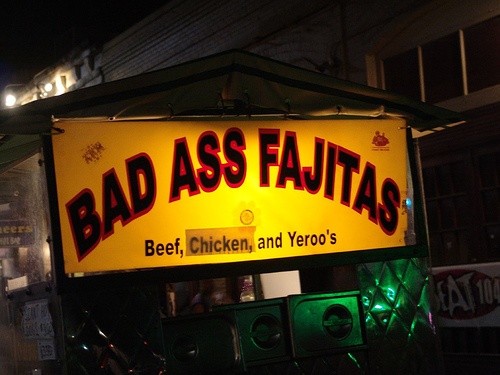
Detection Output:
[2,50,468,375]
